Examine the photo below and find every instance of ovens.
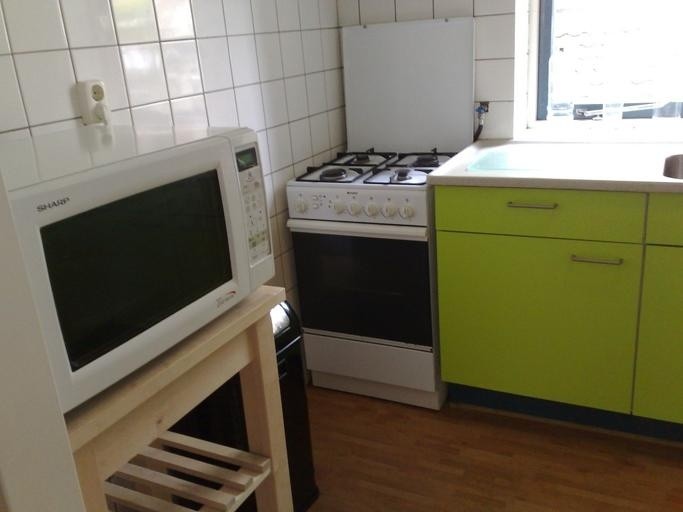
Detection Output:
[286,216,440,393]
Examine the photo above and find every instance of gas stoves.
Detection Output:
[292,148,456,190]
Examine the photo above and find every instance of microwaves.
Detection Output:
[0,127,277,417]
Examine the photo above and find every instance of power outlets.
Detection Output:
[76,79,110,124]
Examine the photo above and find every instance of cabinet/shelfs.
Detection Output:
[64,283,298,511]
[433,187,683,429]
